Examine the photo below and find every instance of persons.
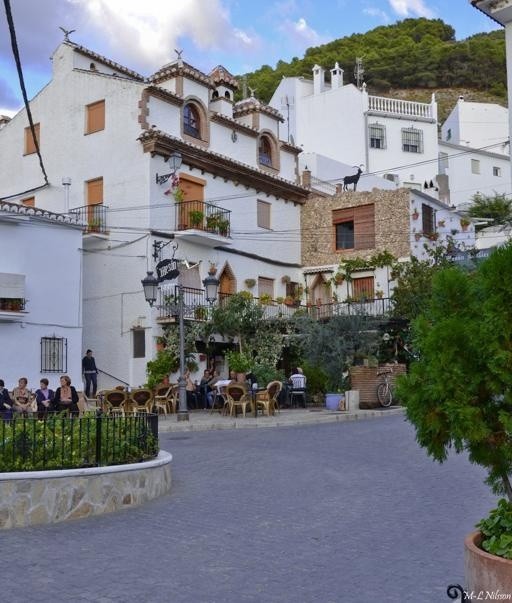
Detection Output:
[12,377,36,418]
[82,349,99,399]
[288,367,306,392]
[184,367,237,410]
[55,375,79,417]
[0,379,13,424]
[35,378,54,421]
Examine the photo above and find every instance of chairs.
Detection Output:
[20,376,307,420]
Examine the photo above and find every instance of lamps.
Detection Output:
[156,148,183,185]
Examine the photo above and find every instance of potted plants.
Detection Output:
[194,305,209,320]
[11,298,21,310]
[217,220,229,237]
[461,217,470,230]
[145,351,178,389]
[285,295,294,305]
[206,216,218,232]
[415,233,422,241]
[428,231,438,240]
[88,217,103,232]
[413,207,418,220]
[189,209,204,229]
[390,240,512,603]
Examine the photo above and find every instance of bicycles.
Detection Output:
[377,368,399,407]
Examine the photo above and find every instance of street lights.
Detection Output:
[141,258,221,423]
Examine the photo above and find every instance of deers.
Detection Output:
[343,163,365,192]
